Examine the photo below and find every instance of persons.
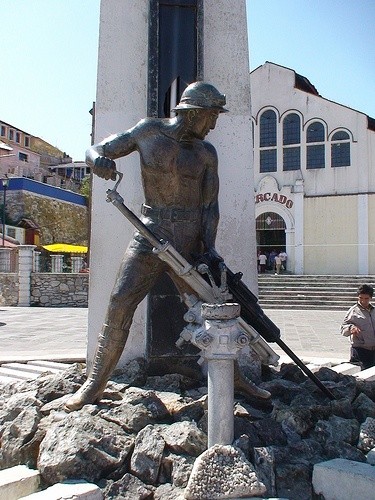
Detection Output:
[340,284,375,371]
[257,248,287,275]
[64,81,271,413]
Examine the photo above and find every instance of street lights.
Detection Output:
[0,173,11,248]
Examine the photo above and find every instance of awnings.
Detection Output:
[43,244,88,253]
